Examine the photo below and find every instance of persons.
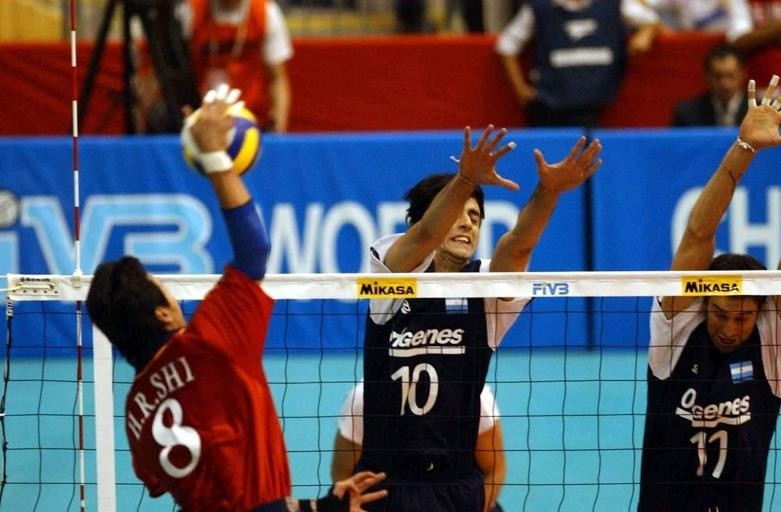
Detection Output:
[351,124,604,512]
[249,470,390,512]
[635,74,781,511]
[672,45,761,129]
[84,83,293,512]
[332,369,508,512]
[392,1,781,39]
[495,2,664,128]
[134,1,294,138]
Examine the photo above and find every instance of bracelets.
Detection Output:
[200,151,235,174]
[457,177,480,188]
[736,139,758,158]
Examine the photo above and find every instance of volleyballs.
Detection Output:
[180,104,261,177]
